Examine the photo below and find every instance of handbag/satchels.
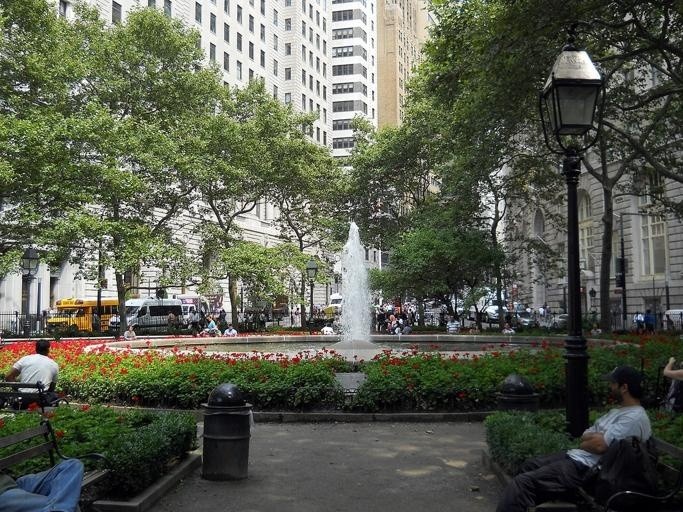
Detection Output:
[583,434,662,512]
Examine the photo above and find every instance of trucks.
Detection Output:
[176,293,223,329]
[244,293,288,322]
[452,298,508,323]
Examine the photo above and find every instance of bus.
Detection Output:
[46,296,119,336]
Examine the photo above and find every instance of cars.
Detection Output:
[505,311,569,330]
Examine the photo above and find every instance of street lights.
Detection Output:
[306,254,319,335]
[19,244,40,314]
[538,20,605,441]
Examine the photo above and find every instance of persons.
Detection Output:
[662,355,682,414]
[495,365,652,511]
[10,299,681,340]
[3,340,58,410]
[0,459,84,511]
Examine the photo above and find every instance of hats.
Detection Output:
[602,365,644,385]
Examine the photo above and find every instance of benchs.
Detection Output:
[571,367,683,512]
[1,380,119,512]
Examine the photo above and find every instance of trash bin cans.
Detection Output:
[200,383,254,482]
[494,373,541,413]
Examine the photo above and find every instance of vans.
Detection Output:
[662,309,683,331]
[328,295,342,315]
[108,297,184,336]
